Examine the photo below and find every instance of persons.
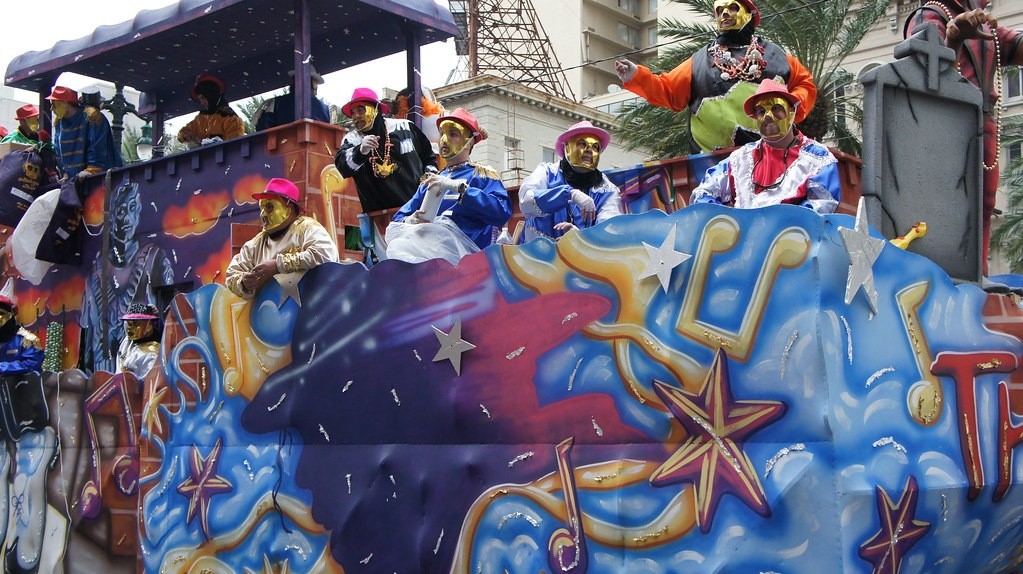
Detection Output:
[0,86,143,176]
[179,64,454,147]
[115,304,162,379]
[689,80,842,218]
[0,295,45,374]
[518,121,623,244]
[334,88,440,268]
[615,0,817,155]
[903,0,1023,293]
[225,178,340,303]
[392,107,513,265]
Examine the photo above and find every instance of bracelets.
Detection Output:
[458,182,466,195]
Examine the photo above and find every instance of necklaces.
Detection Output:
[357,120,394,178]
[922,1,1005,171]
[750,137,800,188]
[708,37,768,81]
[438,160,471,172]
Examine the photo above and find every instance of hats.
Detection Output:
[341,87,389,118]
[287,64,324,84]
[190,73,222,102]
[736,0,760,28]
[252,178,305,214]
[555,121,610,159]
[118,304,160,320]
[0,295,18,316]
[80,87,106,106]
[14,104,39,120]
[44,86,81,103]
[436,108,488,144]
[744,79,800,119]
